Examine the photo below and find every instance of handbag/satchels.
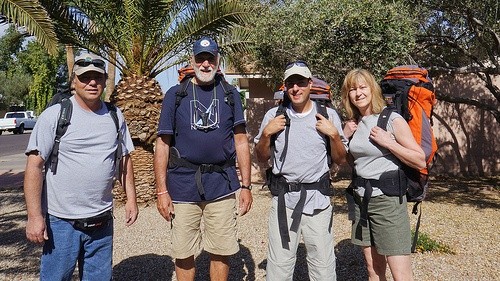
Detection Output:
[60,211,115,233]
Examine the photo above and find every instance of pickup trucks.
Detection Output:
[0,111,37,134]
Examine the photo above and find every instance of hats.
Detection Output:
[284,60,313,81]
[73,53,106,76]
[193,36,218,56]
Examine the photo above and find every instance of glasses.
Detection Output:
[283,62,310,72]
[74,59,106,68]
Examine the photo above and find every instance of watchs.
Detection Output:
[241,184,252,190]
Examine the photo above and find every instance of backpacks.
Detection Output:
[262,78,335,196]
[167,64,234,174]
[44,88,120,187]
[346,65,437,203]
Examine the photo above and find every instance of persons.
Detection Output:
[339,67,426,281]
[152,36,254,281]
[251,60,349,281]
[24,53,139,281]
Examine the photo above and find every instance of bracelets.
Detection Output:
[155,190,168,195]
